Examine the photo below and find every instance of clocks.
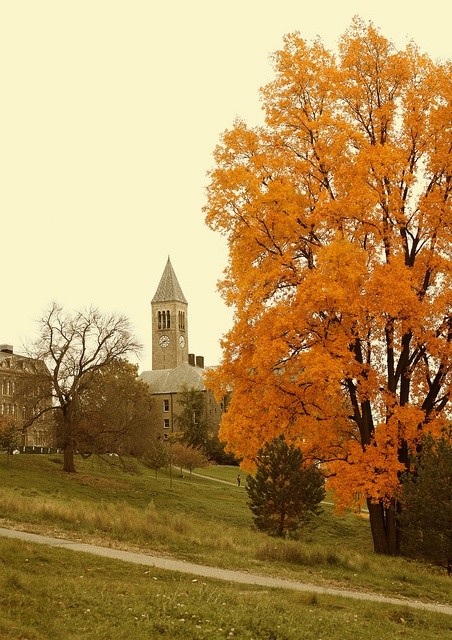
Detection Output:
[179,335,186,348]
[158,335,170,348]
